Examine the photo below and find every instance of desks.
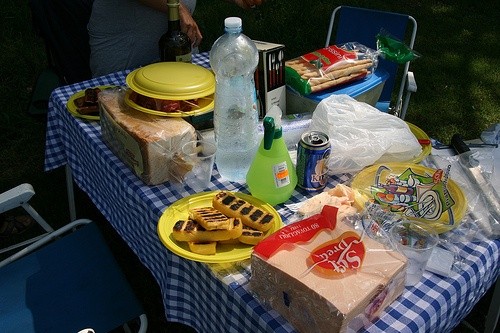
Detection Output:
[44,49,500,333]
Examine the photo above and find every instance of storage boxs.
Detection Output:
[284,61,390,117]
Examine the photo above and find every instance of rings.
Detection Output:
[251,5,256,8]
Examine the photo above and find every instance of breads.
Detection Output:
[97,90,197,185]
[250,218,408,333]
[74,87,103,115]
[171,192,274,255]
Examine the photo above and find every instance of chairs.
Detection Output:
[0,180,164,333]
[325,5,417,118]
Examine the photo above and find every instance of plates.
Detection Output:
[66,85,123,121]
[349,162,468,237]
[124,61,216,118]
[371,121,432,163]
[157,190,283,264]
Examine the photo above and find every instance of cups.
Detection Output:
[390,220,440,287]
[181,141,217,193]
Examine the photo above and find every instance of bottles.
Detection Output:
[208,17,260,186]
[159,1,193,64]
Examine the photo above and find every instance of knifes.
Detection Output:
[451,133,500,220]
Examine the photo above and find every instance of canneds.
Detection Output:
[296,130,332,192]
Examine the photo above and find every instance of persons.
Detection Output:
[88,0,261,79]
